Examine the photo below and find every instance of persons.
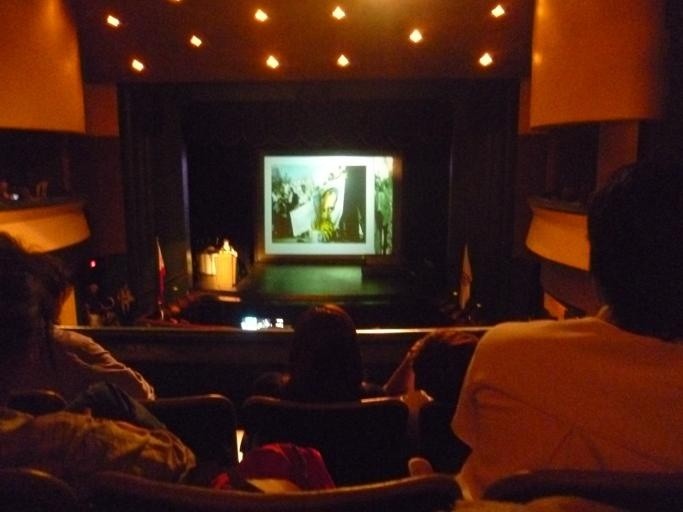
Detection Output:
[272,167,338,242]
[1,232,478,511]
[452,162,683,512]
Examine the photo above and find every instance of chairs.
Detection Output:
[0,373,683,512]
